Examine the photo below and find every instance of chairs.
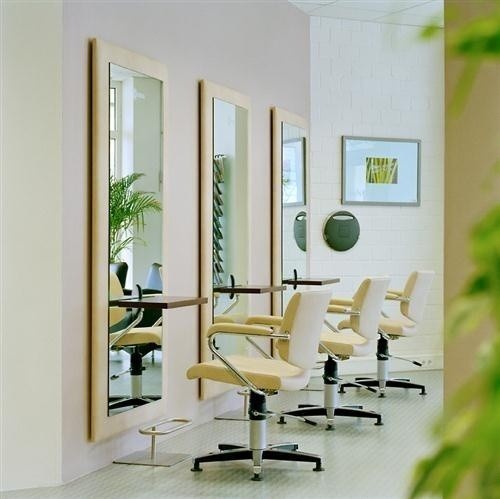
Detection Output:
[109,271,163,409]
[275,274,383,430]
[213,291,242,361]
[186,290,332,481]
[338,270,436,397]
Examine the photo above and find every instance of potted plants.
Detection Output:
[108,172,163,288]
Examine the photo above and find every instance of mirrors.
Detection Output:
[272,106,309,359]
[89,37,168,441]
[200,79,253,400]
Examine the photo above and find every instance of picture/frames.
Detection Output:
[283,137,305,206]
[342,136,421,206]
